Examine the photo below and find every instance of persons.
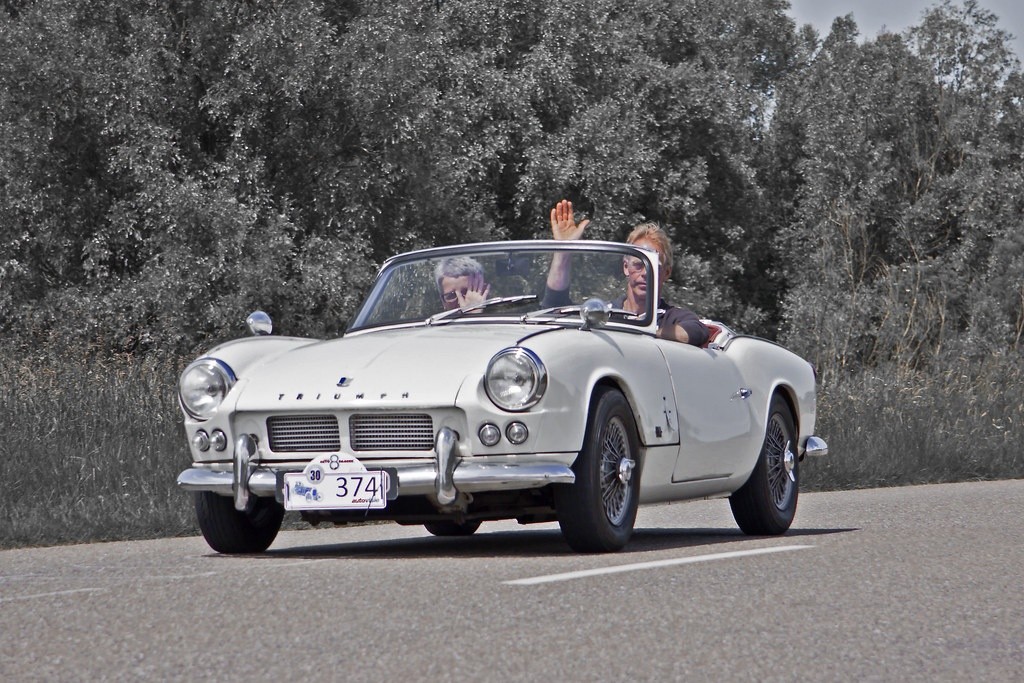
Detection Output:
[433,255,492,313]
[541,199,710,346]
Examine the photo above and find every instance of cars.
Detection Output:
[177,240,829,554]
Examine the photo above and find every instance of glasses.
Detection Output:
[627,259,664,273]
[439,290,468,303]
